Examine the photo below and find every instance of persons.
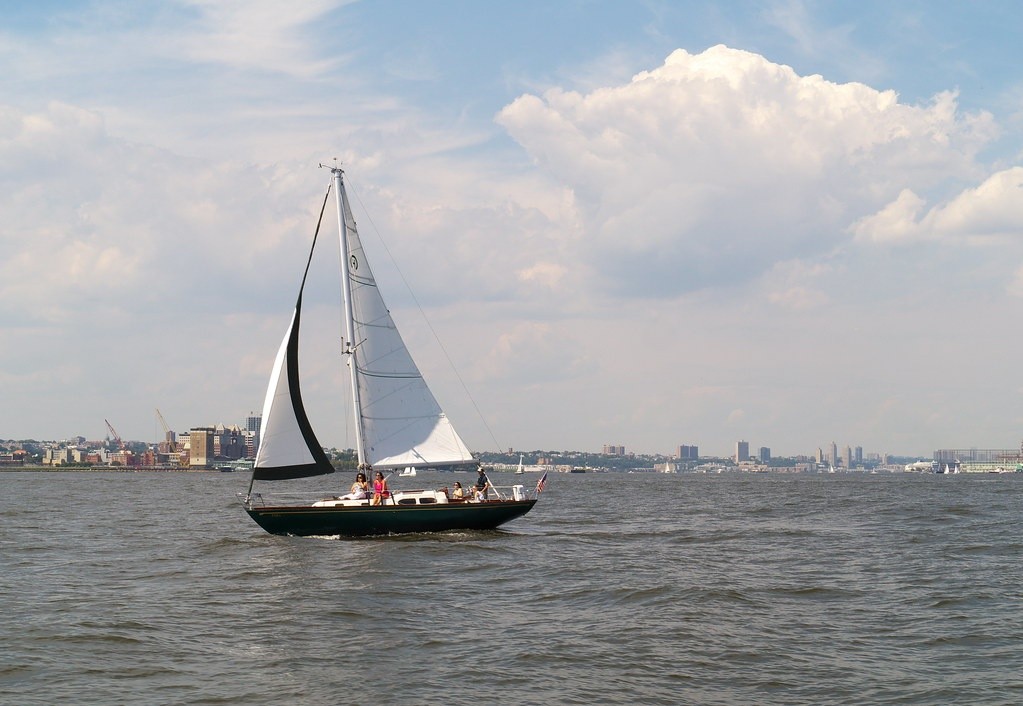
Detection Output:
[333,473,367,499]
[472,486,484,500]
[373,472,389,505]
[453,482,463,499]
[476,468,488,499]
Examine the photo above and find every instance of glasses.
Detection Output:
[454,484,458,486]
[376,475,381,476]
[358,477,362,478]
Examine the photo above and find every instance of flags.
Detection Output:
[537,472,547,492]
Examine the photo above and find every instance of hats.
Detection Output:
[478,468,484,472]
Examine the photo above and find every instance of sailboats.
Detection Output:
[515,456,524,474]
[243,153,548,536]
